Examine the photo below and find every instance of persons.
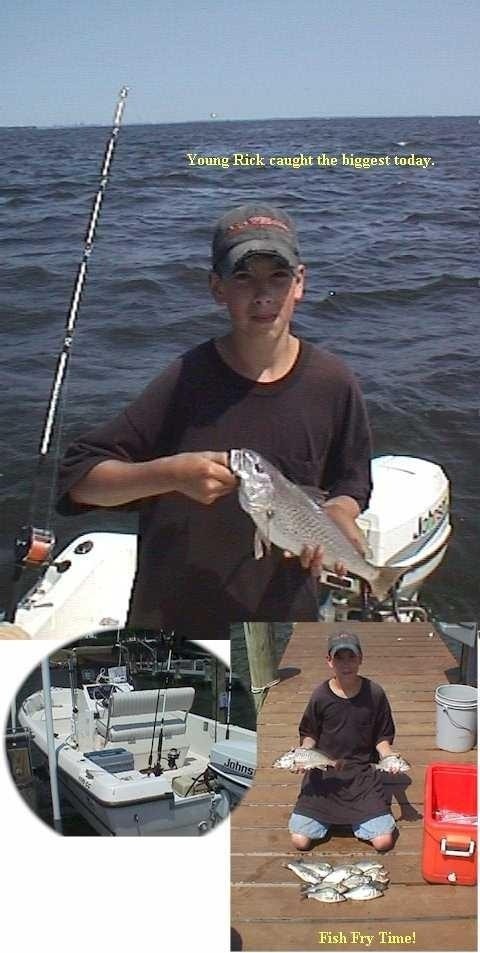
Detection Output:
[52,194,373,639]
[284,628,404,853]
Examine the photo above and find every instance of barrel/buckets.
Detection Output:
[433,685,477,753]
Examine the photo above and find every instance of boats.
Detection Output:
[19,680,259,837]
[2,81,456,637]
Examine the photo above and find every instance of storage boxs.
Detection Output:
[420,764,477,886]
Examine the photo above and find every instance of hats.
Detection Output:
[328,630,361,659]
[211,203,299,278]
